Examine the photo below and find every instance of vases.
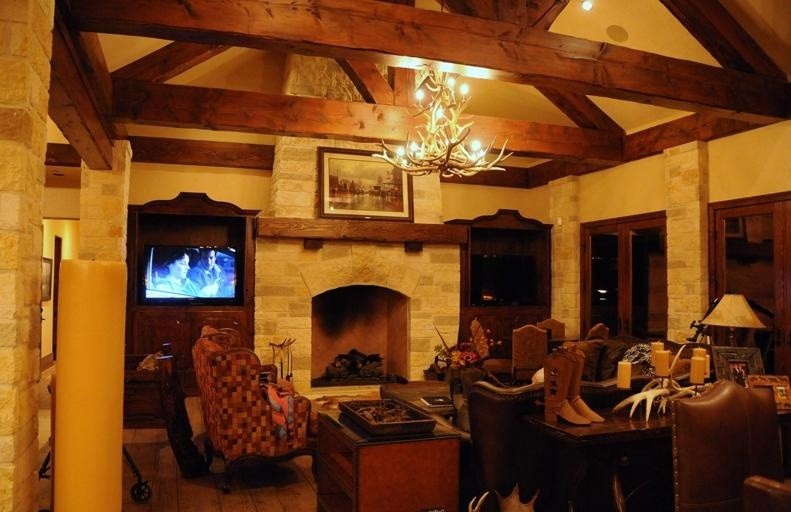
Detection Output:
[460,364,482,399]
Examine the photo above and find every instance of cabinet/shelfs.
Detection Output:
[318,409,462,512]
[125,192,256,377]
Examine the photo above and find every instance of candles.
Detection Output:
[617,339,711,390]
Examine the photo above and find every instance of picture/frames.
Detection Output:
[317,146,415,220]
[711,346,766,391]
[35,257,53,301]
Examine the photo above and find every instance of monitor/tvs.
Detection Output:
[470,255,536,307]
[141,244,238,306]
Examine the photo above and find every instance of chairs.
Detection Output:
[191,325,319,495]
[671,375,791,512]
[482,319,610,386]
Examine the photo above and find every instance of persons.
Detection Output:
[153,250,219,297]
[189,249,228,289]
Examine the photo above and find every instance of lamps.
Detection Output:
[372,60,513,180]
[700,291,767,348]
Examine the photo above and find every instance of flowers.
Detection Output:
[433,321,502,370]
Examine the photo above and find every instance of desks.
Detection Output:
[380,380,467,427]
[523,405,791,512]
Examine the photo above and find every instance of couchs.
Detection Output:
[430,370,745,512]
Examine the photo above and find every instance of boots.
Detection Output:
[544,344,605,426]
